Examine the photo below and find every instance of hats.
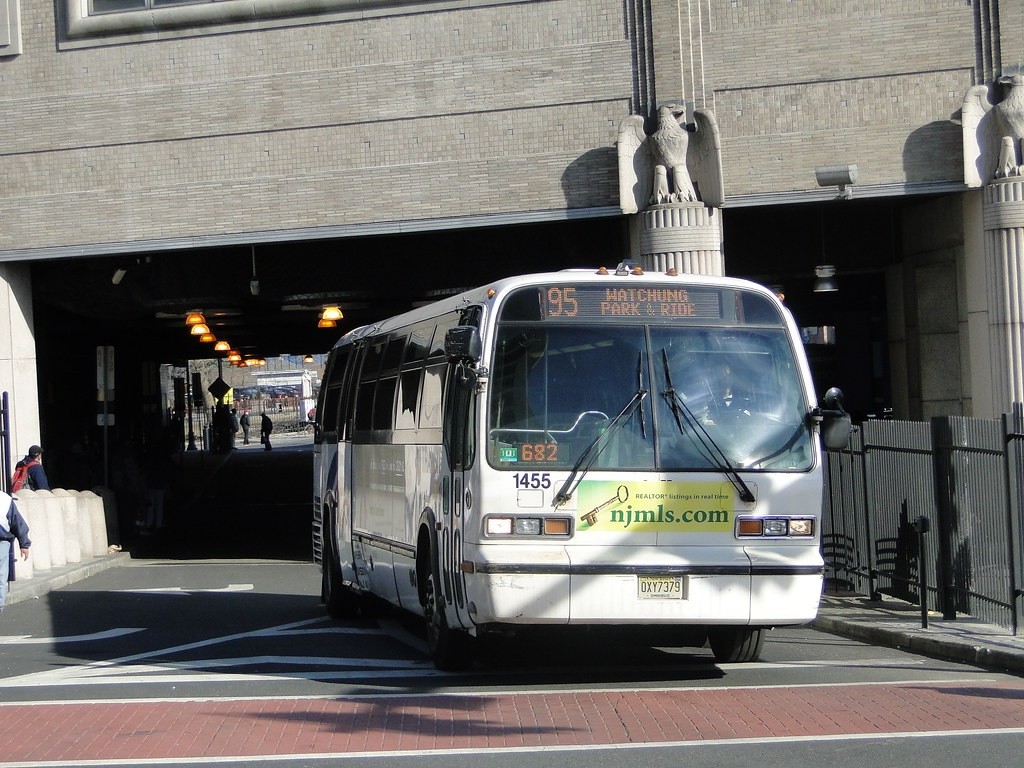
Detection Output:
[29,445,44,454]
[260,413,266,416]
[230,408,236,411]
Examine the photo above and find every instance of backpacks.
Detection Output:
[309,408,316,417]
[12,460,40,493]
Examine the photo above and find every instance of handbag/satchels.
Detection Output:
[261,436,266,444]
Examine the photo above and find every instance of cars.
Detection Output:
[185,384,302,407]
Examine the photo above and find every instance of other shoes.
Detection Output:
[0,605,4,613]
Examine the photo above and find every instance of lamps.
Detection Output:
[322,303,343,320]
[249,246,260,296]
[304,355,314,363]
[318,313,337,328]
[813,264,839,292]
[185,310,265,369]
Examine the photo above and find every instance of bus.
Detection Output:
[299,266,855,664]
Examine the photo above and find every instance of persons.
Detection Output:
[139,453,176,528]
[689,365,749,426]
[240,410,250,446]
[228,408,239,449]
[259,413,273,451]
[16,445,50,493]
[0,491,32,615]
[308,405,317,421]
[215,405,231,454]
[62,442,92,489]
